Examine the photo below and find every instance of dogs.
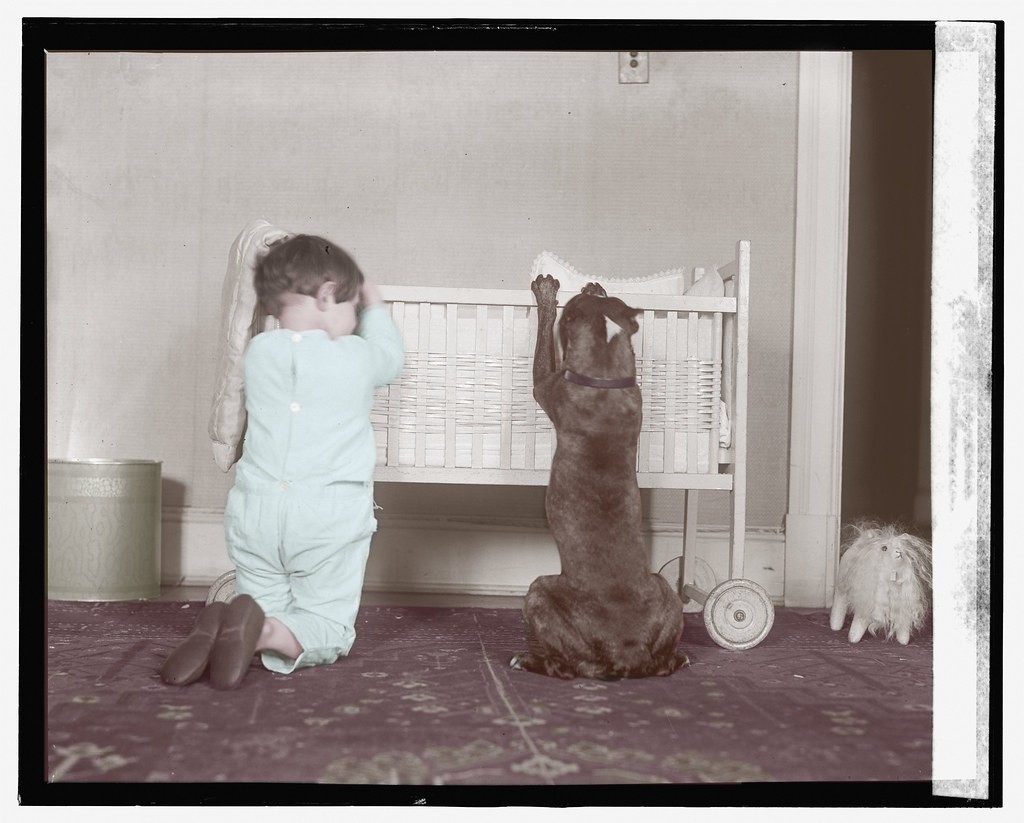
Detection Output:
[512,273,694,679]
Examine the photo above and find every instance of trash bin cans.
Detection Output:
[47,460,163,603]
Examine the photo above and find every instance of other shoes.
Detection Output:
[210,594,265,690]
[160,600,228,686]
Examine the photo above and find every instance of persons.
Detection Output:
[162,235,404,689]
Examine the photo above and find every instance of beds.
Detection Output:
[206,219,774,653]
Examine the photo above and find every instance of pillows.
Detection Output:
[531,251,684,320]
